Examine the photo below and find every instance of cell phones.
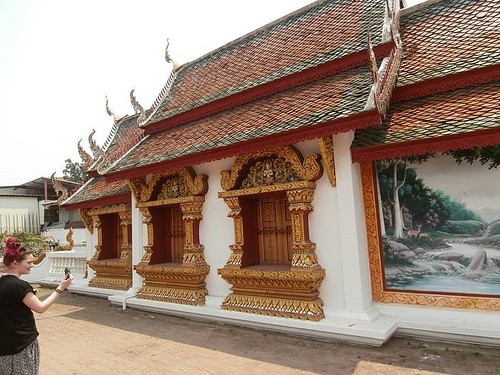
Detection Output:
[64,267,70,279]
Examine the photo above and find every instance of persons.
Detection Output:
[46,218,53,228]
[0,237,75,375]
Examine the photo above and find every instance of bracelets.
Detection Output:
[54,288,63,294]
